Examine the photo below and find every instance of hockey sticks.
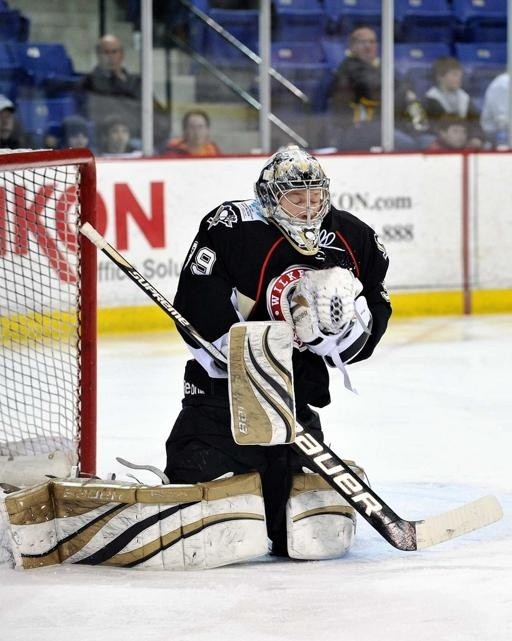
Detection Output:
[79,220,502,551]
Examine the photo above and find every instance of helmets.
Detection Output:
[257,148,331,257]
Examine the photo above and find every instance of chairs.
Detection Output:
[168,0,512,155]
[0,0,161,158]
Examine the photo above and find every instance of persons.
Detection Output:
[1,34,221,155]
[161,144,392,559]
[329,29,511,152]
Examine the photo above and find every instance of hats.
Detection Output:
[1,95,18,113]
